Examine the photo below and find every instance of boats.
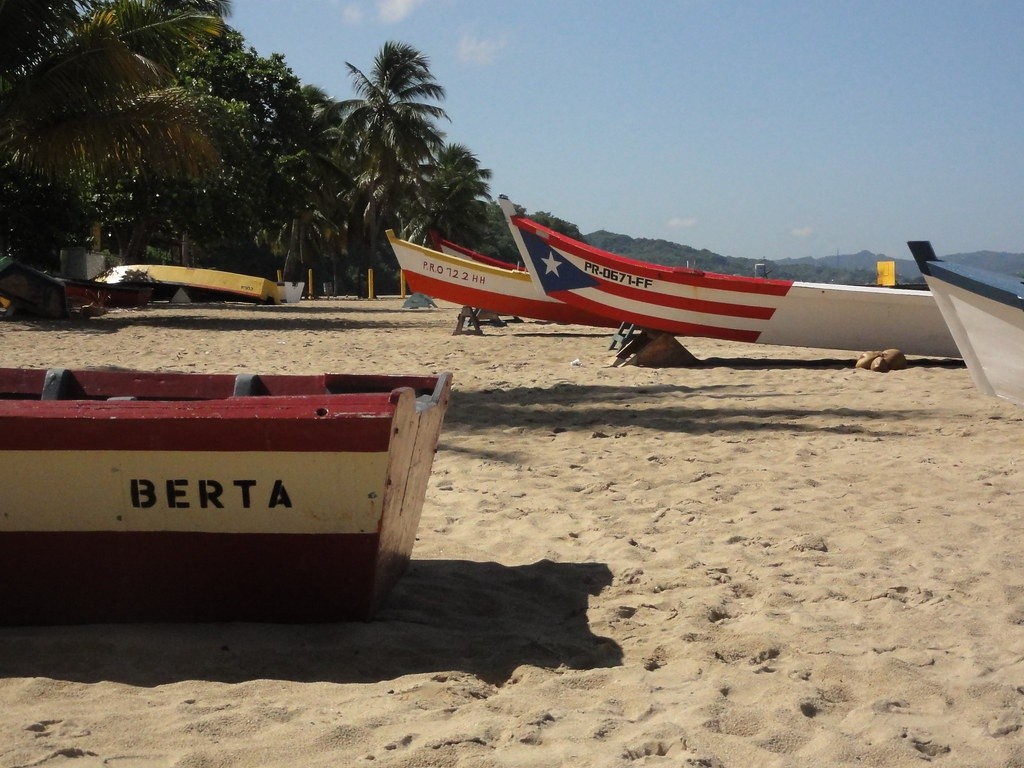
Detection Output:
[427,229,529,272]
[0,368,457,628]
[497,193,965,360]
[0,254,156,323]
[385,229,645,330]
[90,264,284,306]
[907,240,1024,408]
